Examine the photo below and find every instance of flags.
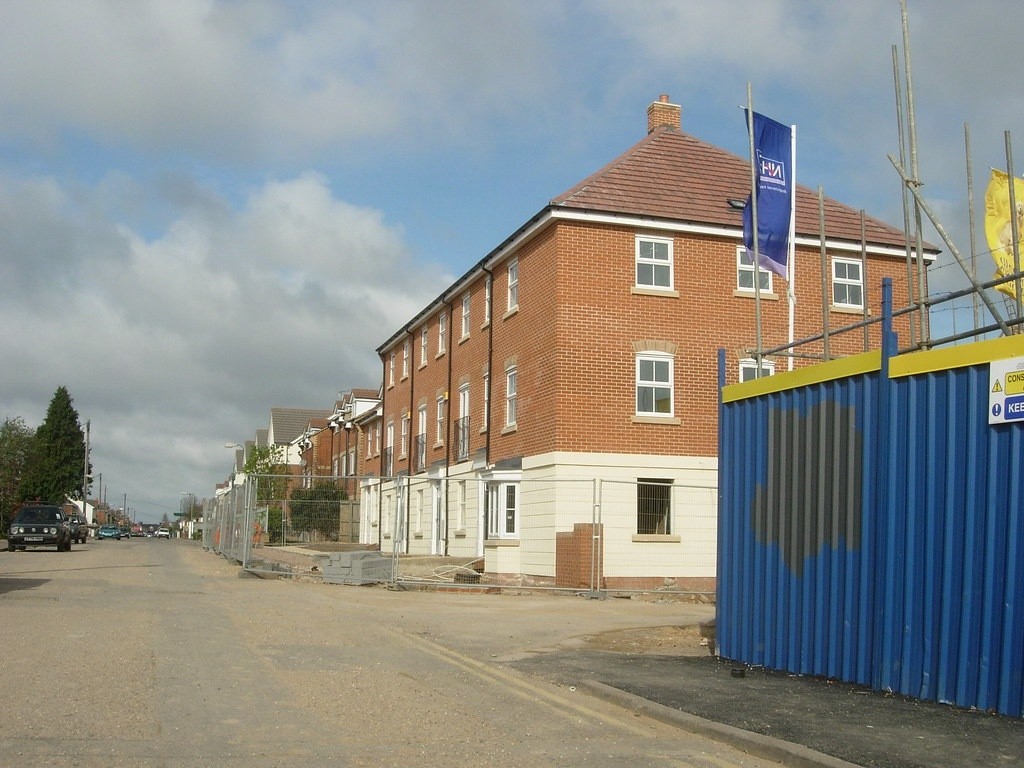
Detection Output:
[985,164,1024,306]
[743,108,790,281]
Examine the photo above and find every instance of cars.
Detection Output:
[118,527,130,539]
[97,523,121,541]
[131,531,158,537]
[157,528,170,540]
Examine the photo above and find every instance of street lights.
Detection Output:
[223,441,246,470]
[181,491,193,539]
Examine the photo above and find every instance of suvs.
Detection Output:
[66,514,89,545]
[7,500,72,552]
